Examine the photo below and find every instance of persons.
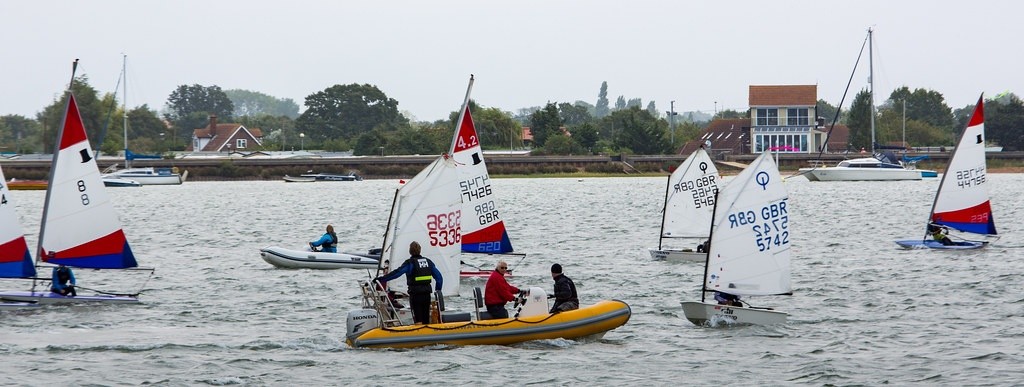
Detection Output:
[932,217,961,245]
[372,241,443,324]
[714,292,742,305]
[550,263,578,312]
[310,223,337,252]
[484,261,524,319]
[52,264,76,296]
[376,266,404,313]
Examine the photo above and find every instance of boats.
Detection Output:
[342,271,632,352]
[282,175,317,182]
[259,243,383,270]
[5,178,48,191]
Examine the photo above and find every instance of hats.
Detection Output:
[551,263,562,274]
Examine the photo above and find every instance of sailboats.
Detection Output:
[359,151,463,325]
[893,91,1000,254]
[795,25,931,182]
[93,53,184,188]
[0,55,156,309]
[439,72,528,279]
[678,149,796,328]
[647,141,726,264]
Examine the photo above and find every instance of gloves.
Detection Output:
[547,294,555,299]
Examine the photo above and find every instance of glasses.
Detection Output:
[501,266,507,269]
